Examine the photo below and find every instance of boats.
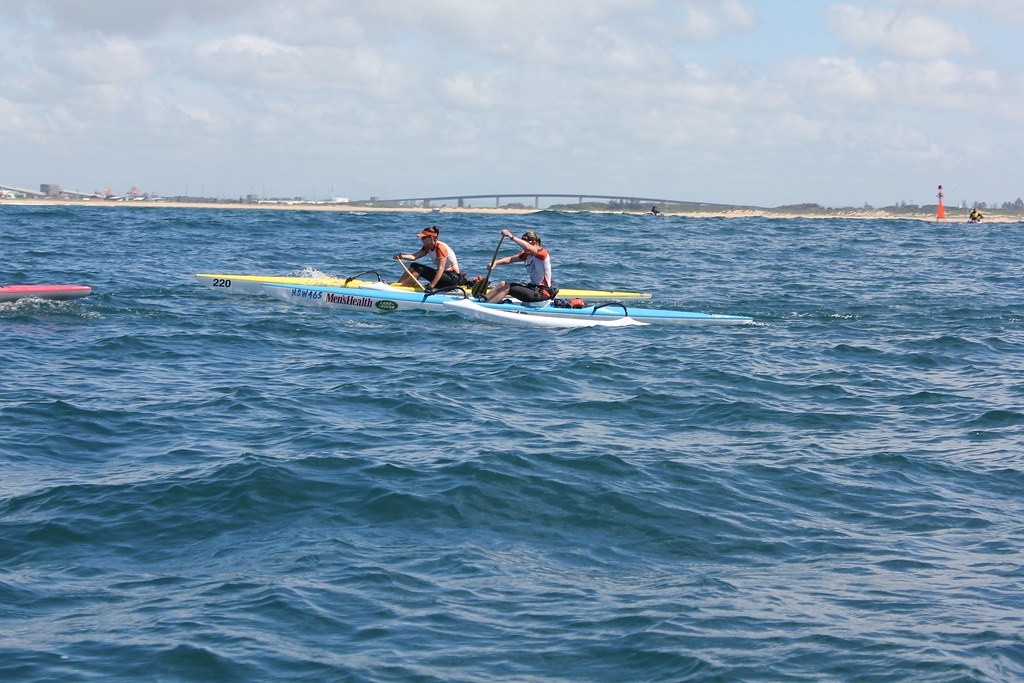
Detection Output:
[967,215,982,224]
[253,278,758,326]
[1,280,93,303]
[188,272,431,304]
[436,298,651,331]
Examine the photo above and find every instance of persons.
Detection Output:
[652,205,661,216]
[391,224,462,295]
[479,230,556,305]
[969,208,984,221]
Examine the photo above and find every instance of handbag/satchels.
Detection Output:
[449,270,467,285]
[550,280,559,300]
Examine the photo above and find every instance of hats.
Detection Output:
[416,230,438,237]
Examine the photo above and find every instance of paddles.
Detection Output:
[472,236,505,298]
[396,255,434,293]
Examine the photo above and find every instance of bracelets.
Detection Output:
[509,233,514,241]
